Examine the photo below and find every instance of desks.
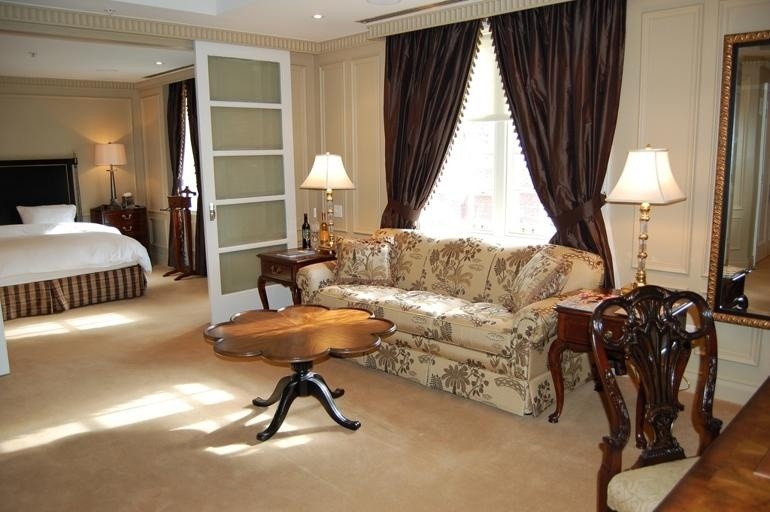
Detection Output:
[654,375,770,512]
[718,265,752,314]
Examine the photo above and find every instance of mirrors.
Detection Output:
[704,29,770,331]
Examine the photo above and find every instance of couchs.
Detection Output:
[296,229,606,418]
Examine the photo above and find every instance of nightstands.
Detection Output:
[90,206,150,256]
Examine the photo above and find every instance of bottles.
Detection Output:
[318,212,331,251]
[302,213,312,250]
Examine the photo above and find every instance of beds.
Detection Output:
[1,157,152,322]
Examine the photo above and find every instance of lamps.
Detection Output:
[300,151,356,248]
[94,142,127,209]
[604,143,687,296]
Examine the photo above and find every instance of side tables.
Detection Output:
[256,247,337,310]
[547,288,695,423]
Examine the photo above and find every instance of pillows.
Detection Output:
[369,228,439,290]
[418,236,501,303]
[501,248,572,313]
[484,243,605,315]
[16,204,77,224]
[331,234,398,287]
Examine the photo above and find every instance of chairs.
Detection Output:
[589,285,723,512]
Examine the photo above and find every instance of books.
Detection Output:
[276,250,315,257]
